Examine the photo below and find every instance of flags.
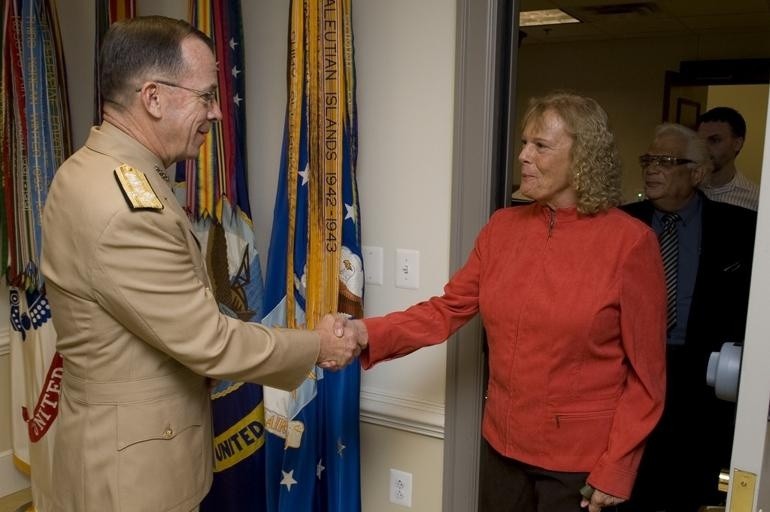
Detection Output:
[261,0,365,512]
[0,0,74,512]
[93,0,134,125]
[172,0,266,512]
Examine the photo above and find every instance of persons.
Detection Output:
[39,13,362,512]
[314,89,667,512]
[617,121,758,512]
[696,106,760,211]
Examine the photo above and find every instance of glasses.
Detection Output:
[637,154,696,169]
[155,78,219,108]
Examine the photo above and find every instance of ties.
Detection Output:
[656,212,682,329]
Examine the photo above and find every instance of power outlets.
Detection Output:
[387,467,412,508]
